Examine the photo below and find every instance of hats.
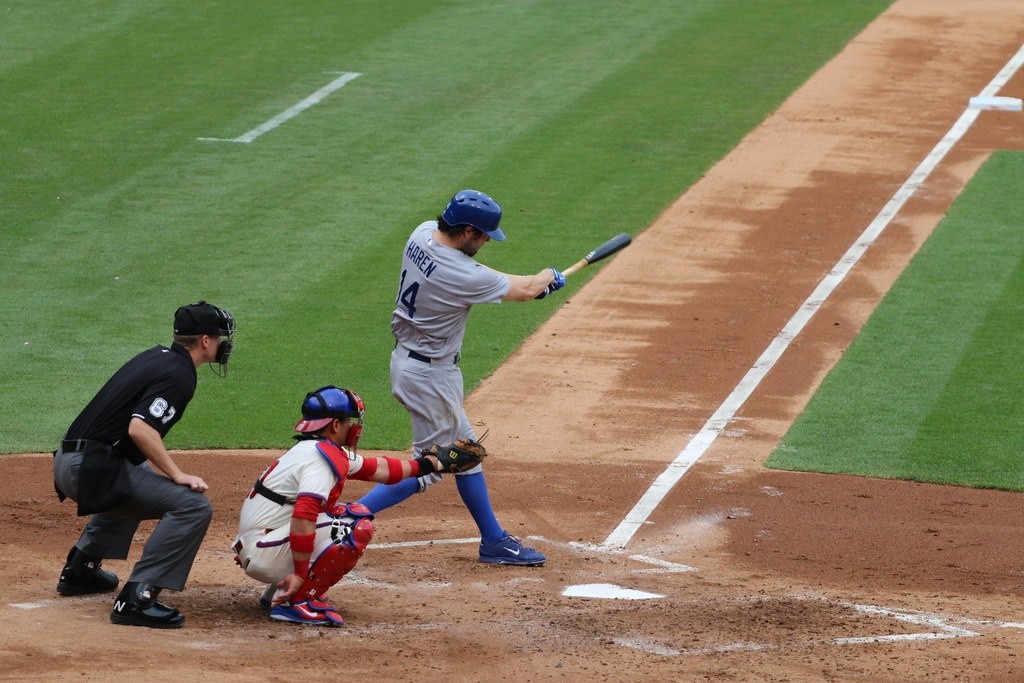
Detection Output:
[173,305,232,339]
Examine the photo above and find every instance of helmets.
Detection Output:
[441,190,506,240]
[294,386,359,432]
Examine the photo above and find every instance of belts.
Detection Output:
[233,529,274,553]
[60,439,85,452]
[395,340,460,364]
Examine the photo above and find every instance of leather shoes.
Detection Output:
[110,582,185,628]
[56,545,119,595]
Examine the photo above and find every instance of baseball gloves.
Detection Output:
[419,427,490,479]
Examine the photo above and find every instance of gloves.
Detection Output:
[535,267,565,300]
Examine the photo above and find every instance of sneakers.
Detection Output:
[478,530,547,565]
[265,595,342,625]
[259,586,330,607]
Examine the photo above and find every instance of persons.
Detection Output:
[47,303,236,628]
[231,383,489,625]
[355,189,568,568]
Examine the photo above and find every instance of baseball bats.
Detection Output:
[560,229,633,277]
